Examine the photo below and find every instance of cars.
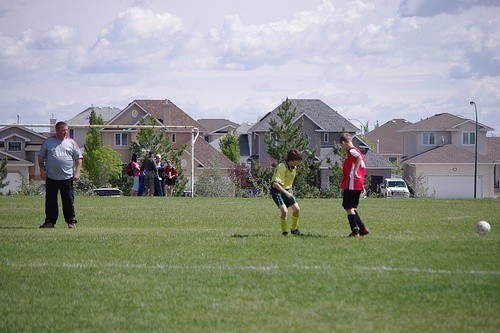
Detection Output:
[86,187,123,197]
[379,178,409,199]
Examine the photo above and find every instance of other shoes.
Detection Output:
[67,220,76,228]
[39,222,54,228]
[347,229,359,237]
[359,227,369,236]
[282,231,288,236]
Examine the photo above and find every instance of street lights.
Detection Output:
[470,101,478,198]
[348,117,366,128]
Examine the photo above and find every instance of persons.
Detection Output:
[270,149,305,236]
[37,122,83,228]
[338,133,370,238]
[130,151,179,197]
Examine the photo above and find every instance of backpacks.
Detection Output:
[126,162,133,176]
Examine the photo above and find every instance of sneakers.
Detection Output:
[291,229,303,235]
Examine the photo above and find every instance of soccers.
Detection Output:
[476,221,490,235]
